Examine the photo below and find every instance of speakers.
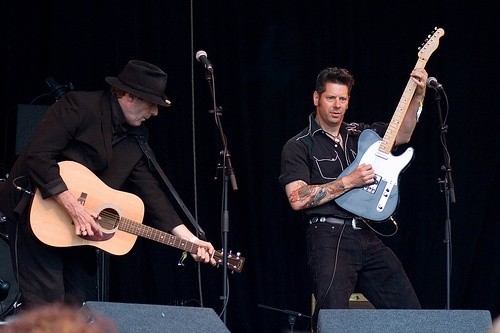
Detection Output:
[317,309,493,333]
[79,301,231,333]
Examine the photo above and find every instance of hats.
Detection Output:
[105,59,172,106]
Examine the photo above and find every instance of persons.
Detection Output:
[0,60,217,333]
[279,68,428,333]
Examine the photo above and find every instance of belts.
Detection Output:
[307,216,372,229]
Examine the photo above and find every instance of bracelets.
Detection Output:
[414,96,424,121]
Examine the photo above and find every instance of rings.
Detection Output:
[96,214,99,219]
[81,229,86,232]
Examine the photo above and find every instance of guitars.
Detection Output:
[334,26,445,221]
[29,161,246,274]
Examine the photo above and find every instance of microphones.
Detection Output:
[196,50,213,74]
[427,77,442,88]
